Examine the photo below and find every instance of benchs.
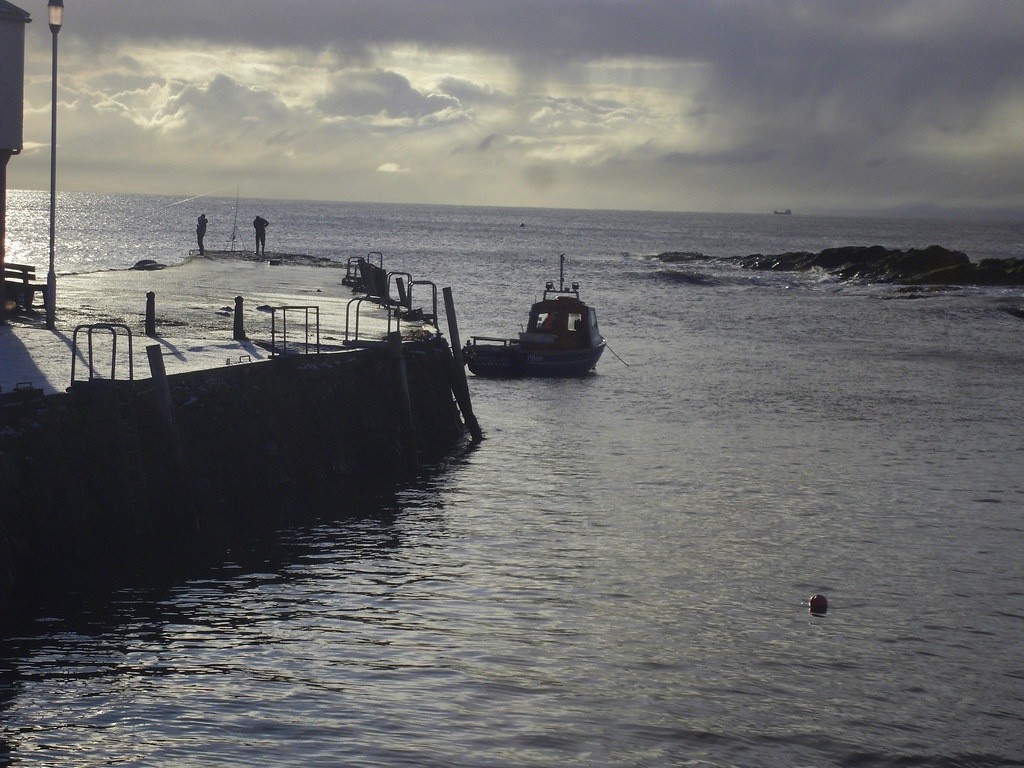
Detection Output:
[3,261,48,312]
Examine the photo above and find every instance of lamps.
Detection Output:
[545,282,555,291]
[571,282,579,291]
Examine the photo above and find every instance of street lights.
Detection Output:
[41,0,67,330]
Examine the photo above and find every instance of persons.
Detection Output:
[196,214,208,256]
[253,216,270,255]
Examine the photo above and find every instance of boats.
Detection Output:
[462,252,608,374]
